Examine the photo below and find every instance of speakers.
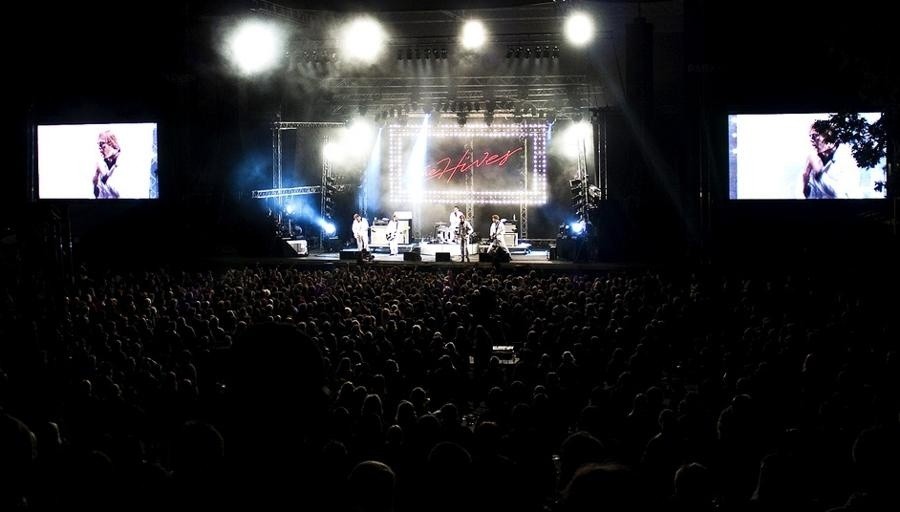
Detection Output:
[355,251,371,259]
[436,253,450,262]
[340,251,355,260]
[479,253,493,262]
[493,253,509,262]
[404,252,421,262]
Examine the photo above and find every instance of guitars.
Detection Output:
[386,226,411,241]
[487,233,501,244]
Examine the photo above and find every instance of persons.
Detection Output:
[0,207,900,512]
[91,131,137,199]
[802,121,860,198]
